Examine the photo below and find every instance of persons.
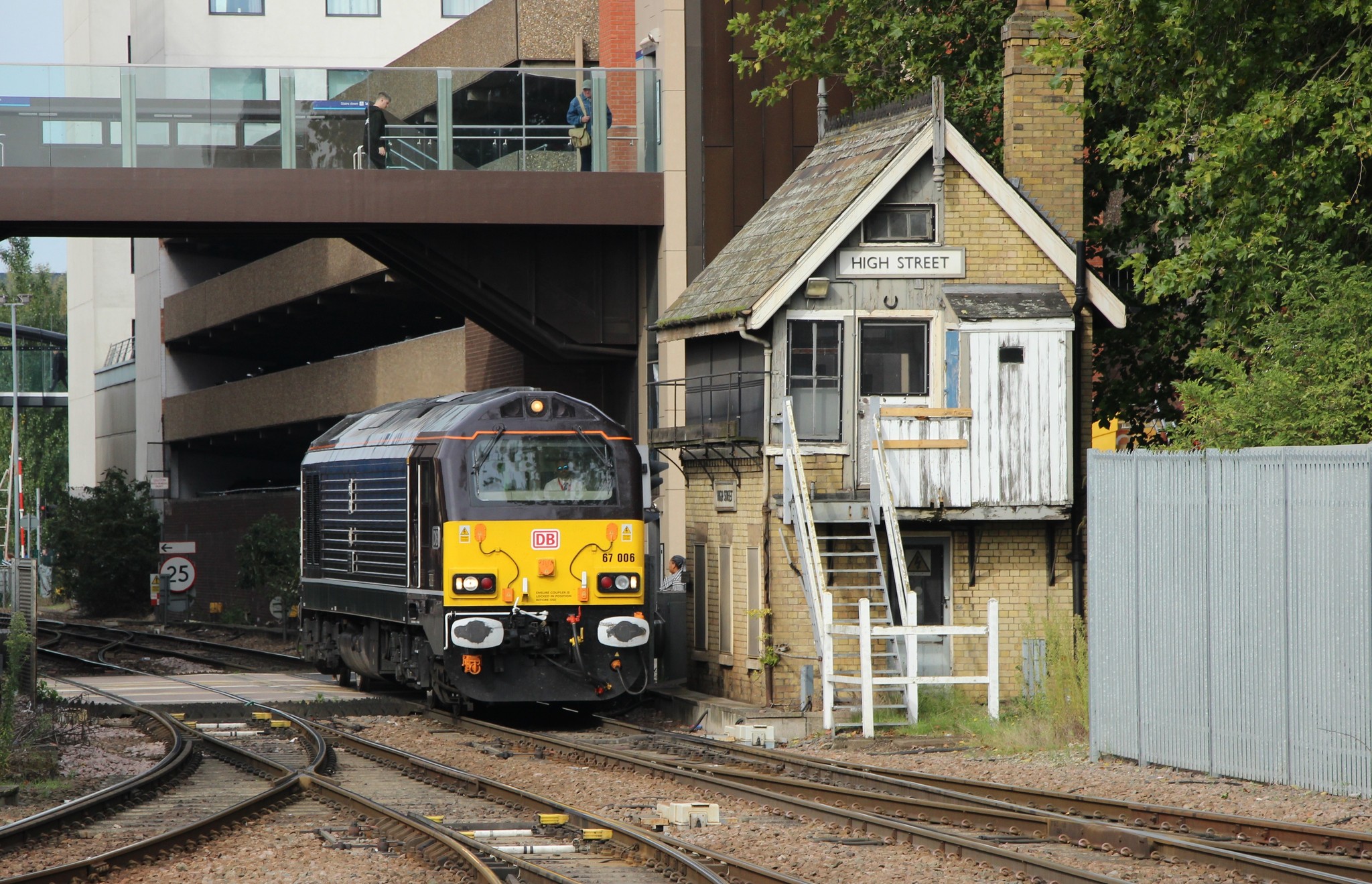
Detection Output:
[658,555,687,593]
[567,79,612,172]
[363,92,394,169]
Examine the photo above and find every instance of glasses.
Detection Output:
[558,465,569,470]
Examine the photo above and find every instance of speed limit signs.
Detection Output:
[159,555,197,593]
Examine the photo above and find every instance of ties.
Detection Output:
[564,482,568,490]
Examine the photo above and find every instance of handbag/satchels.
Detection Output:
[568,127,591,148]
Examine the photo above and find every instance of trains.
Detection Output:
[299,386,654,726]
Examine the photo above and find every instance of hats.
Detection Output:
[582,79,591,89]
